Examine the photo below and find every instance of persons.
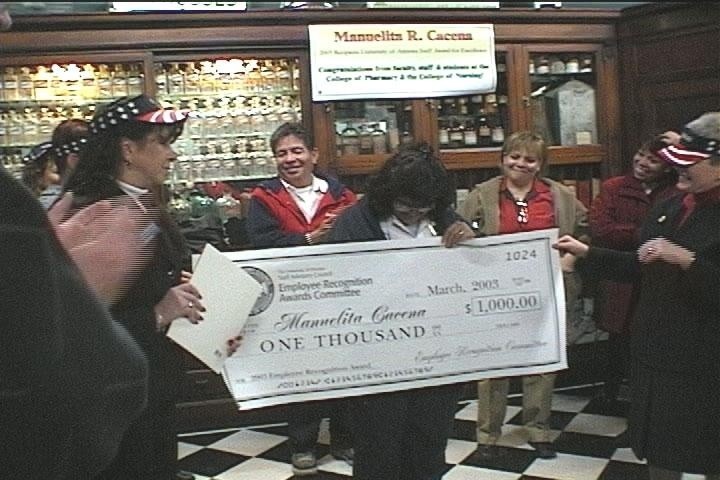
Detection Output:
[0,164,148,478]
[552,111,719,479]
[39,118,93,212]
[43,92,243,480]
[459,128,593,461]
[247,123,363,475]
[18,136,61,199]
[587,127,690,414]
[327,135,487,479]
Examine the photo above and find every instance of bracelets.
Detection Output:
[304,233,314,245]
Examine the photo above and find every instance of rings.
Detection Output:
[458,229,465,234]
[646,246,655,253]
[186,300,196,309]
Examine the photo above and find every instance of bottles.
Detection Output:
[154,60,302,183]
[0,153,23,183]
[334,106,414,156]
[1,103,97,146]
[438,95,508,150]
[167,188,251,236]
[1,57,147,99]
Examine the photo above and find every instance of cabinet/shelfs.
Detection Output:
[627,2,720,146]
[0,14,625,208]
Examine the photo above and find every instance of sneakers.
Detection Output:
[527,439,557,459]
[290,449,319,478]
[478,443,499,462]
[331,447,356,467]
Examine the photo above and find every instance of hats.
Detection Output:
[656,128,719,168]
[86,94,190,136]
